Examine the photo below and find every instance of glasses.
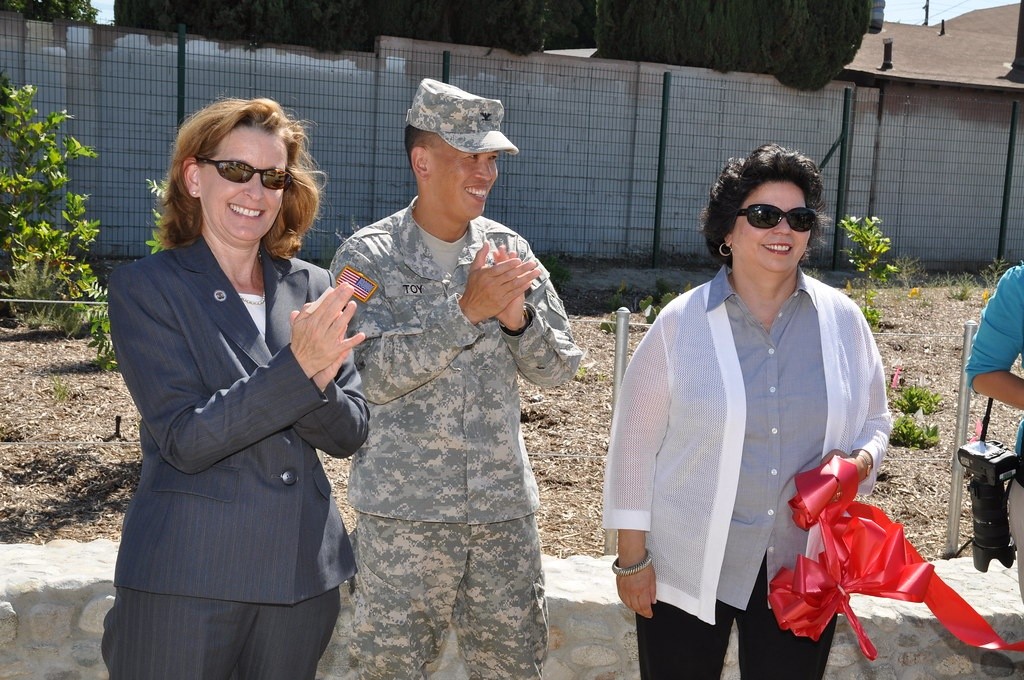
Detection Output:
[737,204,817,232]
[195,156,293,192]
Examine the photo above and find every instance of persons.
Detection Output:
[332,79,584,679]
[101,96,371,680]
[965,264,1024,600]
[601,143,890,680]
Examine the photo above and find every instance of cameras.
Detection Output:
[957,441,1024,573]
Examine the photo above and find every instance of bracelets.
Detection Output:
[853,452,871,476]
[612,549,651,576]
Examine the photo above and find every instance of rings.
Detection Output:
[836,491,841,499]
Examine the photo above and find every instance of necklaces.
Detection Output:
[243,292,265,305]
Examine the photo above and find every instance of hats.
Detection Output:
[405,78,520,156]
